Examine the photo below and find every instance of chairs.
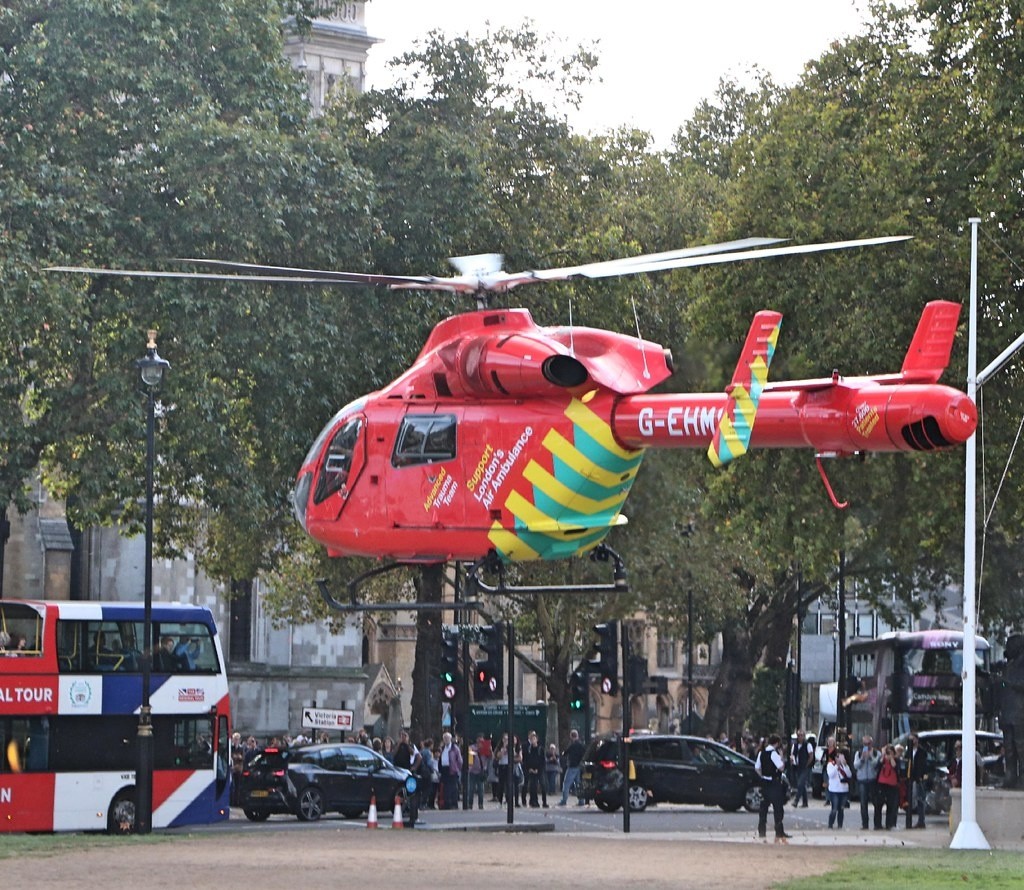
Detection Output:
[153,652,164,672]
[180,652,191,671]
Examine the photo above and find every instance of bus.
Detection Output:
[0,598,233,836]
[844,627,993,759]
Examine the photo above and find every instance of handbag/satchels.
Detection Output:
[479,772,488,781]
[488,758,498,783]
[513,764,523,785]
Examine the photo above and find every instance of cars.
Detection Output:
[237,741,424,823]
[881,729,1009,816]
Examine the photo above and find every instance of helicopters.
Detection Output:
[35,203,977,612]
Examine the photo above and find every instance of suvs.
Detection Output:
[571,734,793,813]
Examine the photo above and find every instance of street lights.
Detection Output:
[131,327,171,833]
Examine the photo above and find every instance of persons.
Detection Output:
[0,631,41,657]
[854,733,927,830]
[946,740,1005,788]
[754,735,793,837]
[91,631,117,663]
[160,637,176,671]
[348,731,559,810]
[690,744,707,763]
[327,445,353,490]
[826,751,852,830]
[705,729,850,808]
[174,636,200,670]
[197,732,329,808]
[394,430,427,466]
[558,729,584,806]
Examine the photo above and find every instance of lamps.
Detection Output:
[395,677,403,692]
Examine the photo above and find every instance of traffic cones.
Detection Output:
[391,795,403,830]
[366,794,379,829]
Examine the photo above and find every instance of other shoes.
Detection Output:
[792,804,798,807]
[823,801,830,806]
[801,805,808,808]
[515,804,521,807]
[776,832,792,838]
[542,804,549,807]
[915,823,926,829]
[759,830,765,837]
[558,802,566,806]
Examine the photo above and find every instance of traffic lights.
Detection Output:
[649,674,664,694]
[572,672,586,710]
[591,623,610,677]
[476,625,496,683]
[438,638,458,687]
